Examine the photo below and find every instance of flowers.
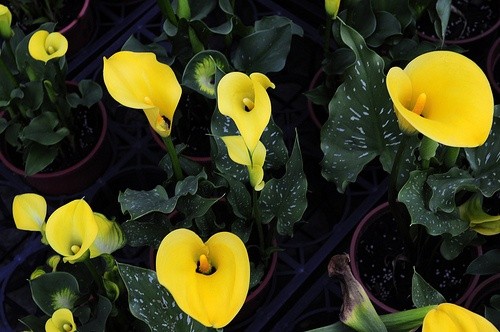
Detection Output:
[0,0,500,332]
